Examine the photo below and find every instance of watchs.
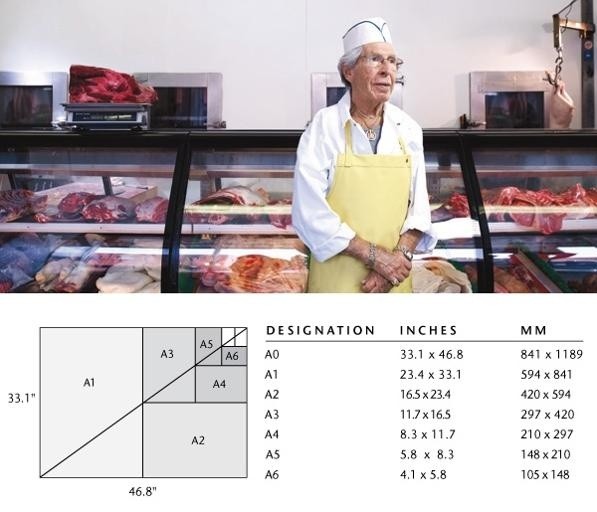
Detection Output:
[393,246,413,260]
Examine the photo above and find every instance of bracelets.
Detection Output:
[368,242,376,271]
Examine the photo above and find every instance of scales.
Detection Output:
[57,103,153,132]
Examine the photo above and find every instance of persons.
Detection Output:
[290,16,438,293]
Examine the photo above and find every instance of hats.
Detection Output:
[342,17,393,54]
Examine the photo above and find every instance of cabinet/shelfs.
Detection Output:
[1,128,597,293]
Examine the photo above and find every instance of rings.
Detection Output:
[392,279,398,285]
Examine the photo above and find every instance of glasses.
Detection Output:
[358,54,397,67]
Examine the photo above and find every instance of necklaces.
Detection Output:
[356,109,383,140]
